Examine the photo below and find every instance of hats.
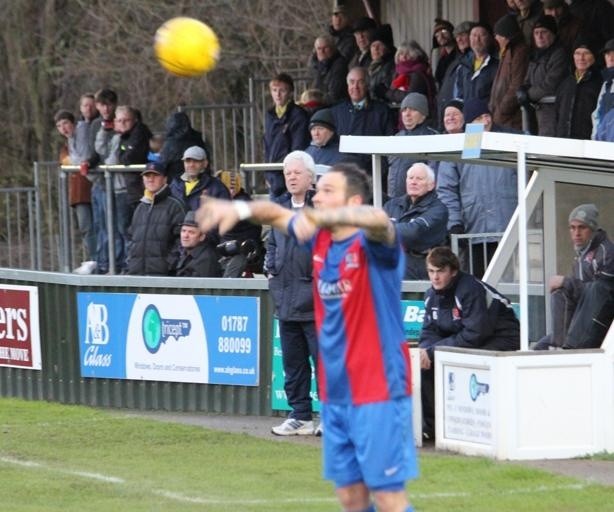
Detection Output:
[352,17,394,49]
[307,108,338,131]
[442,95,491,124]
[570,203,600,232]
[182,146,206,162]
[533,15,558,35]
[400,92,429,116]
[495,14,520,40]
[600,39,614,53]
[141,162,166,176]
[452,20,478,36]
[432,19,455,48]
[177,211,199,227]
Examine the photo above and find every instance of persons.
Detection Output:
[190,160,423,510]
[413,244,521,444]
[526,201,614,352]
[260,149,322,436]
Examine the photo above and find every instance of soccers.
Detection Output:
[155,17,221,75]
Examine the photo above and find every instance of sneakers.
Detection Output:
[271,418,312,437]
[74,260,98,275]
